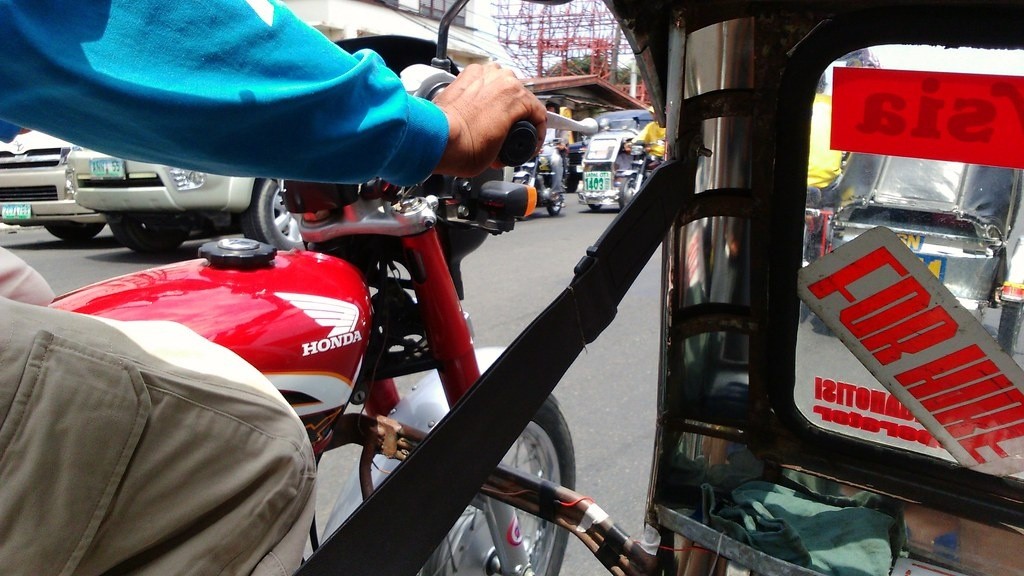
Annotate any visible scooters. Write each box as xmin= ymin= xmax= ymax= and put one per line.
xmin=804 ymin=186 xmax=835 ymax=266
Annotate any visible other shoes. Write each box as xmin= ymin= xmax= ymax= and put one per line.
xmin=551 ymin=192 xmax=559 ymax=201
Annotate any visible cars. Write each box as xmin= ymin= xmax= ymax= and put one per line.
xmin=828 ymin=149 xmax=1017 ymax=332
xmin=1 ymin=129 xmax=108 ymax=244
xmin=63 ymin=135 xmax=312 ymax=255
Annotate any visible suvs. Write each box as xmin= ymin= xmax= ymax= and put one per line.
xmin=564 ymin=109 xmax=656 ymax=194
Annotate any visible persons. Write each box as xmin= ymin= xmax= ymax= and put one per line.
xmin=624 ymin=106 xmax=667 ymax=160
xmin=0 ymin=0 xmax=553 ymax=576
xmin=541 ymin=104 xmax=570 ymax=200
xmin=803 ymin=47 xmax=885 ymax=266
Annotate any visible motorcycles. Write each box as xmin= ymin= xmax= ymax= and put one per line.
xmin=508 ymin=137 xmax=571 ymax=216
xmin=576 ymin=131 xmax=656 ymax=213
xmin=30 ymin=2 xmax=582 ymax=576
